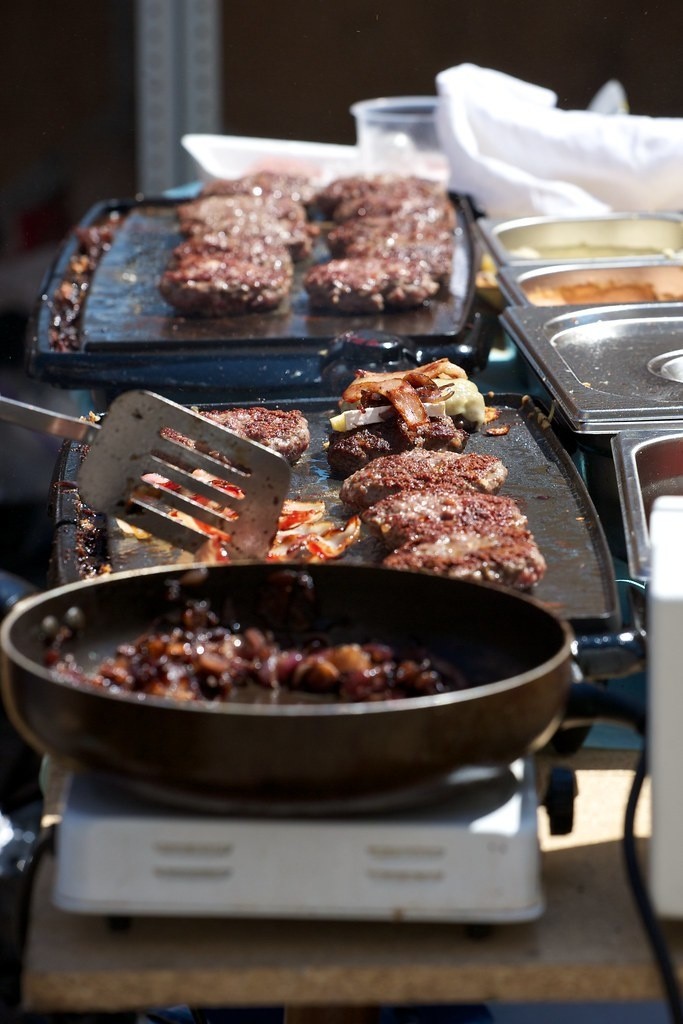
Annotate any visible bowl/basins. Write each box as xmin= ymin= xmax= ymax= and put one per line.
xmin=345 ymin=97 xmax=450 ymax=179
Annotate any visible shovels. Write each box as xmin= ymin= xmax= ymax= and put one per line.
xmin=0 ymin=390 xmax=291 ymax=560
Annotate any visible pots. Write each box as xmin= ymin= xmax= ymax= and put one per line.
xmin=1 ymin=560 xmax=576 ymax=805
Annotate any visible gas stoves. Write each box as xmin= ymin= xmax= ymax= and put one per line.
xmin=23 ymin=757 xmax=582 ymax=932
